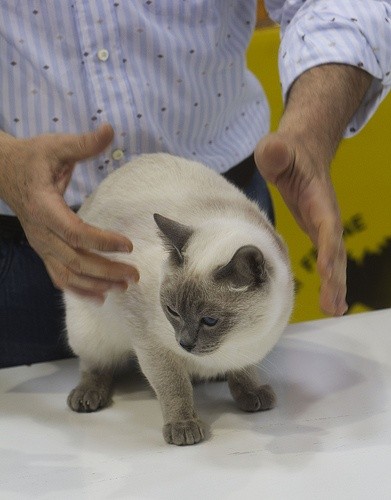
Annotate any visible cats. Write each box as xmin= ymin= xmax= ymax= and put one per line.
xmin=56 ymin=151 xmax=295 ymax=446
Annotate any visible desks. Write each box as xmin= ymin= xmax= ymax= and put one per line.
xmin=0 ymin=305 xmax=391 ymax=500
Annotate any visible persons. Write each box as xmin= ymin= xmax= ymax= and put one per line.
xmin=0 ymin=1 xmax=391 ymax=369
